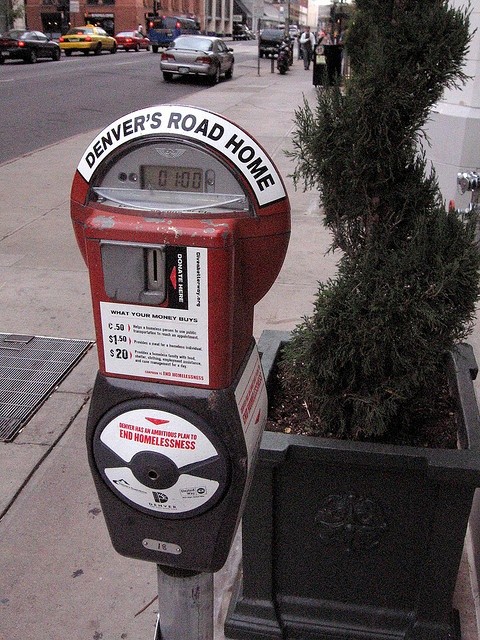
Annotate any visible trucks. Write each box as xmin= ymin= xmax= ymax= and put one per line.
xmin=149 ymin=15 xmax=201 ymax=53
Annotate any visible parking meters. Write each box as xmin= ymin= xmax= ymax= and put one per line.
xmin=71 ymin=105 xmax=290 ymax=640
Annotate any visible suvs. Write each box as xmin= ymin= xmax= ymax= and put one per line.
xmin=258 ymin=29 xmax=295 ymax=58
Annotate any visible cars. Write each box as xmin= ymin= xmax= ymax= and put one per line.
xmin=160 ymin=34 xmax=234 ymax=83
xmin=114 ymin=30 xmax=150 ymax=51
xmin=59 ymin=24 xmax=118 ymax=57
xmin=0 ymin=29 xmax=61 ymax=64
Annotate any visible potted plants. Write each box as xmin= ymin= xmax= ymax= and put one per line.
xmin=222 ymin=0 xmax=478 ymax=640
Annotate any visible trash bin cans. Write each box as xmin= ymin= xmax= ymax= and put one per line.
xmin=312 ymin=44 xmax=342 ymax=87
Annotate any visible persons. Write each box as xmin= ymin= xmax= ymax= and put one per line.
xmin=297 ymin=28 xmax=304 ymax=60
xmin=138 ymin=23 xmax=143 ymax=34
xmin=299 ymin=26 xmax=316 ymax=70
xmin=318 ymin=31 xmax=333 ymax=45
xmin=232 ymin=21 xmax=239 ymax=41
